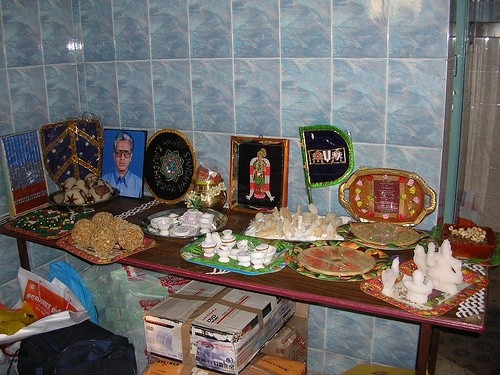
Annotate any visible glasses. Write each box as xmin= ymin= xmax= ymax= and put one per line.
xmin=114 ymin=151 xmax=133 ymax=159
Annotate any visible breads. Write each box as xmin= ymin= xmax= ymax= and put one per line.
xmin=71 ymin=212 xmax=144 ymax=252
xmin=62 ymin=173 xmax=112 ymax=204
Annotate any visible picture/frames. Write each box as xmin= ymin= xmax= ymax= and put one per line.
xmin=230 ymin=135 xmax=290 ymax=215
xmin=101 ymin=129 xmax=147 ymax=200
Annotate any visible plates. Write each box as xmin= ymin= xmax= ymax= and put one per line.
xmin=56 ymin=235 xmax=157 ymax=265
xmin=140 ymin=206 xmax=229 ymax=239
xmin=47 ymin=187 xmax=121 ymax=207
xmin=360 ymin=258 xmax=490 ymax=319
xmin=283 ymin=240 xmax=392 ymax=283
xmin=179 ymin=230 xmax=294 ymax=276
xmin=336 ymin=221 xmax=436 ymax=251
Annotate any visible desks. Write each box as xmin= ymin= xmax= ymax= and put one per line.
xmin=0 ymin=197 xmax=491 ymax=375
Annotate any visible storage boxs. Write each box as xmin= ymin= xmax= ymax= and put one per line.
xmin=140 ymin=280 xmax=310 ymax=375
xmin=340 ymin=363 xmax=415 ymax=375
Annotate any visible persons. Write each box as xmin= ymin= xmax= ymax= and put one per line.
xmin=102 ymin=132 xmax=142 ymax=198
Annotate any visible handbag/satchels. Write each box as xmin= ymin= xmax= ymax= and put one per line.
xmin=7 ymin=319 xmax=138 ymax=375
xmin=0 ymin=261 xmax=99 ymax=345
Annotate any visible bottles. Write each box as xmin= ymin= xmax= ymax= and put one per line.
xmin=192 ymin=181 xmax=227 ymax=210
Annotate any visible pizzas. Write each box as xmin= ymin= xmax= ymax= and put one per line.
xmin=351 ymin=222 xmax=420 ymax=246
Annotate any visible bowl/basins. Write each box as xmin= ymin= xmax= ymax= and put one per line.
xmin=441 ymin=223 xmax=496 ymax=259
